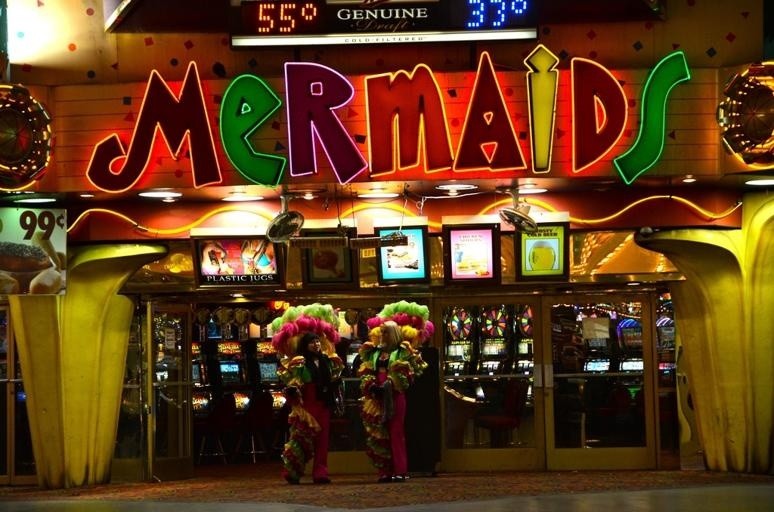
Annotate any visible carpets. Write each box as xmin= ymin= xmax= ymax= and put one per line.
xmin=0 ymin=470 xmax=773 ymax=511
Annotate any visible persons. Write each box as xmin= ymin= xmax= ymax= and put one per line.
xmin=282 ymin=335 xmax=342 ymax=485
xmin=359 ymin=320 xmax=408 ymax=482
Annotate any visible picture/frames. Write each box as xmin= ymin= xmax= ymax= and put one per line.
xmin=441 ymin=222 xmax=503 ymax=286
xmin=373 ymin=225 xmax=432 ymax=286
xmin=298 ymin=227 xmax=362 ymax=290
xmin=514 ymin=221 xmax=570 ymax=286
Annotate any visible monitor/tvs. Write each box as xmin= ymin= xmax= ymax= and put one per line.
xmin=446 ymin=338 xmax=676 ymax=375
xmin=220 ymin=362 xmax=241 ymax=385
xmin=192 ymin=364 xmax=201 ymax=382
xmin=258 ymin=363 xmax=280 ymax=383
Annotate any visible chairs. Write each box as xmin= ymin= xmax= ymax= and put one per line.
xmin=473 ymin=378 xmax=528 ymax=449
xmin=194 ymin=391 xmax=292 ymax=466
xmin=598 ymin=387 xmax=676 ymax=447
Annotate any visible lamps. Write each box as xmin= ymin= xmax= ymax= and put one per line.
xmin=289 ymin=191 xmax=407 ymax=250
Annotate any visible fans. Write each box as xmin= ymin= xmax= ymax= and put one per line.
xmin=267 ymin=195 xmax=304 ymax=243
xmin=494 ymin=188 xmax=538 ymax=236
